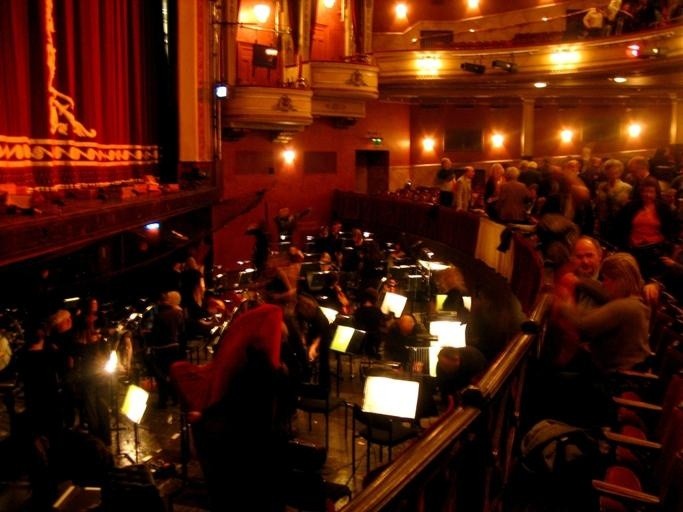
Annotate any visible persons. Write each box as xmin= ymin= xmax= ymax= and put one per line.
xmin=621 ymin=1 xmax=662 ymax=34
xmin=585 ymin=4 xmax=636 ymax=40
xmin=0 ymin=257 xmax=245 ymax=510
xmin=244 ymin=142 xmax=682 ymax=512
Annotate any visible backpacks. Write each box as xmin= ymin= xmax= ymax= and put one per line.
xmin=518 ymin=416 xmax=618 ymax=512
